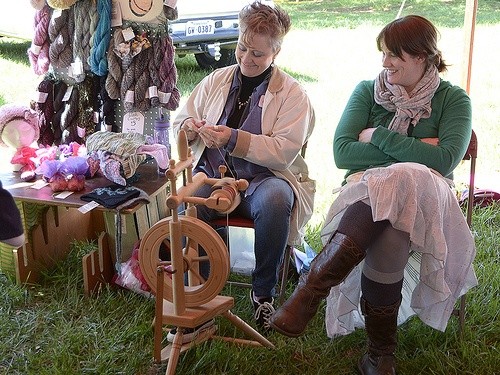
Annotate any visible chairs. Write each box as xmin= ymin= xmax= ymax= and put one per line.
xmin=448 ymin=128 xmax=479 ymax=340
xmin=204 ymin=138 xmax=309 ymax=303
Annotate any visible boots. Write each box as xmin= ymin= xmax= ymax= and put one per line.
xmin=268 ymin=230 xmax=367 ymax=338
xmin=360 ymin=294 xmax=403 ymax=375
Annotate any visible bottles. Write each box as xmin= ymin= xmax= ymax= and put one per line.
xmin=154 ymin=116 xmax=169 ymax=160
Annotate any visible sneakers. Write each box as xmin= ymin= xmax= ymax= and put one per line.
xmin=166 ymin=317 xmax=216 ymax=344
xmin=250 ymin=289 xmax=275 ymax=338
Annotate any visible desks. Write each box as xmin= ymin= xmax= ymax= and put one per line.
xmin=0 ymin=162 xmax=185 ymax=299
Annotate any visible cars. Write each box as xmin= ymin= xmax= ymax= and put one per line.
xmin=0 ymin=0 xmax=274 ymax=77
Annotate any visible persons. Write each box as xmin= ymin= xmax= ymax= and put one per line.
xmin=0 ymin=180 xmax=25 ymax=247
xmin=269 ymin=14 xmax=478 ymax=375
xmin=173 ymin=1 xmax=316 ymax=336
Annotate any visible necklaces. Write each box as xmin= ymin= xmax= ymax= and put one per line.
xmin=238 ymin=93 xmax=251 ymax=110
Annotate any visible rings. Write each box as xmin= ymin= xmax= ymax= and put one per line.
xmin=214 ymin=125 xmax=218 ymax=129
